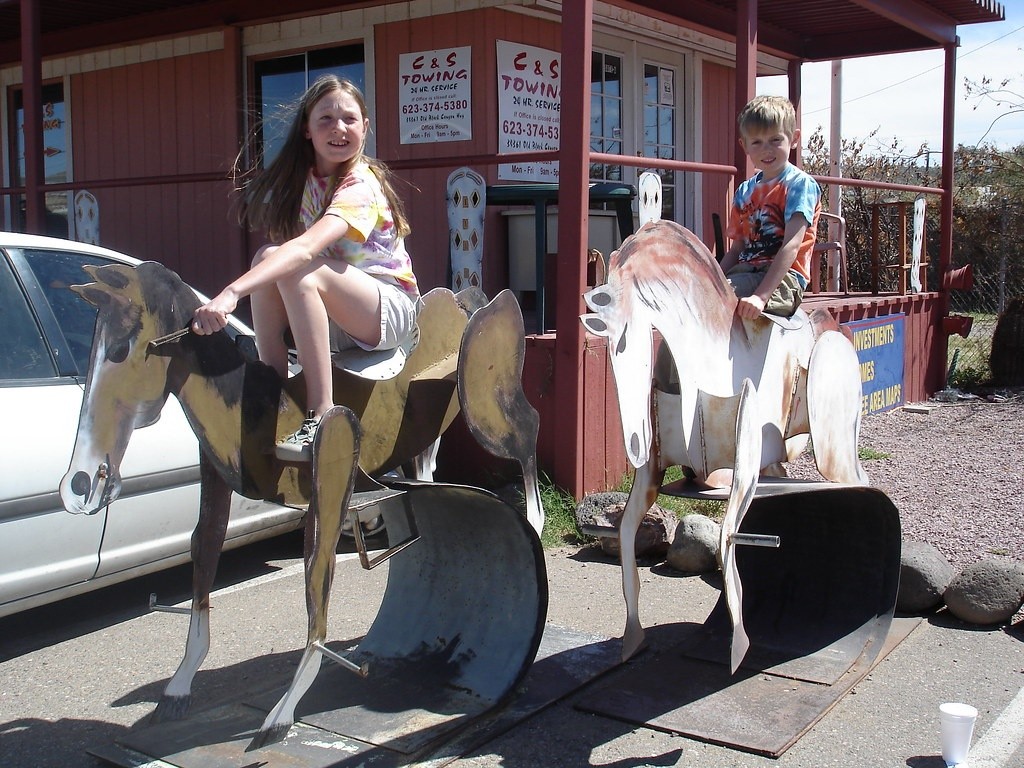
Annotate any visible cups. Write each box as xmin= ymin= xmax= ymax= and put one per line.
xmin=939 ymin=703 xmax=977 ymax=763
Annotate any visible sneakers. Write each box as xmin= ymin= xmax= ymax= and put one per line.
xmin=276 ymin=409 xmax=319 ymax=463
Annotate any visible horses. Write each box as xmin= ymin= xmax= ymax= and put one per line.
xmin=57 ymin=259 xmax=545 ymax=752
xmin=577 ymin=218 xmax=870 ymax=676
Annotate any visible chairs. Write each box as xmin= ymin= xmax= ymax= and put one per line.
xmin=810 ymin=211 xmax=848 ymax=295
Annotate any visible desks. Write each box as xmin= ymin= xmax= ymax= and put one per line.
xmin=485 ymin=181 xmax=634 ymax=320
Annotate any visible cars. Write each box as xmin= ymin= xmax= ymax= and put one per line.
xmin=0 ymin=234 xmax=310 ymax=619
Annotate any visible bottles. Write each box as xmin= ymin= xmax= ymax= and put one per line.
xmin=934 ymin=390 xmax=958 ymax=402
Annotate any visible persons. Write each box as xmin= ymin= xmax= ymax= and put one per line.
xmin=719 ymin=95 xmax=824 ymax=322
xmin=191 ymin=77 xmax=425 ymax=463
